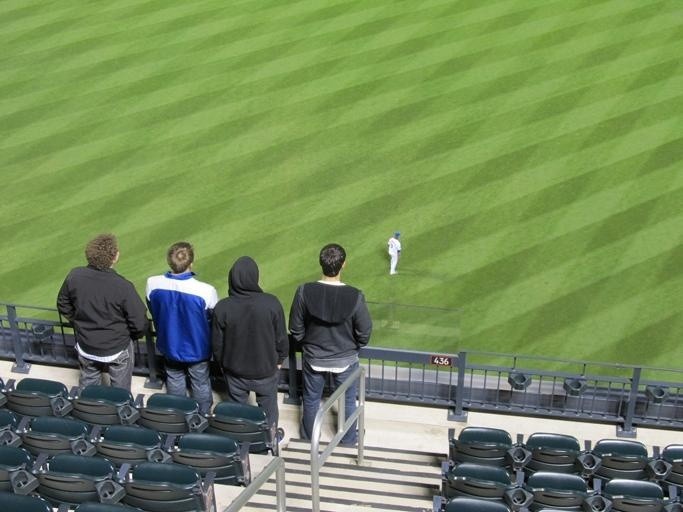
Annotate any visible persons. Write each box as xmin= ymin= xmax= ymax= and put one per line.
xmin=53 ymin=231 xmax=149 ymax=403
xmin=141 ymin=239 xmax=220 ymax=416
xmin=386 ymin=231 xmax=401 ymax=275
xmin=286 ymin=242 xmax=373 ymax=446
xmin=208 ymin=255 xmax=290 ymax=450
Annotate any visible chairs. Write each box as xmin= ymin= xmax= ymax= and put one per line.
xmin=434 ymin=426 xmax=683 ymax=512
xmin=0 ymin=376 xmax=280 ymax=512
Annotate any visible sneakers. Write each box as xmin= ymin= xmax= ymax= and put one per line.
xmin=356 ymin=428 xmax=365 ymax=443
xmin=275 ymin=428 xmax=285 ymax=443
